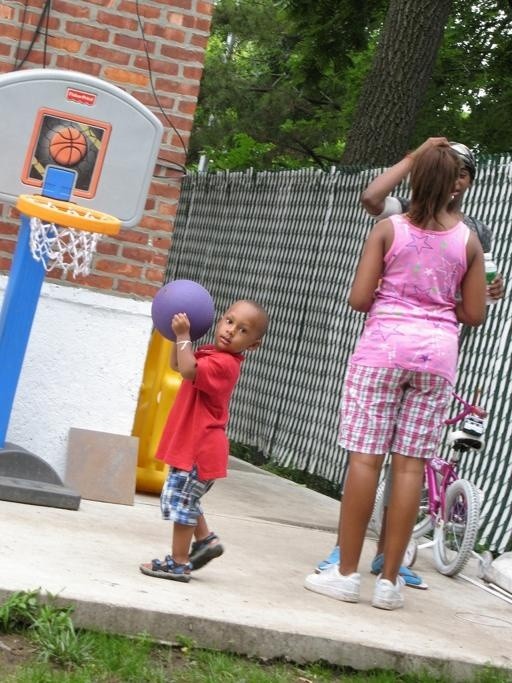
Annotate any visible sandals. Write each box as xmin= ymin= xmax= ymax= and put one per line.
xmin=188 ymin=530 xmax=224 ymax=570
xmin=139 ymin=552 xmax=194 ymax=582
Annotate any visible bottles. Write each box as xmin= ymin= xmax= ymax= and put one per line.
xmin=483 ymin=253 xmax=496 ymax=305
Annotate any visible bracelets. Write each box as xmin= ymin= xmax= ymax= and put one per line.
xmin=176 ymin=341 xmax=192 ymax=351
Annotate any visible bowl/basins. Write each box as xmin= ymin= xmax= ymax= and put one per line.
xmin=446 ymin=141 xmax=475 ymax=168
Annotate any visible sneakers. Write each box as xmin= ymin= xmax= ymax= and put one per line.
xmin=303 ymin=563 xmax=362 ymax=604
xmin=370 ymin=574 xmax=407 ymax=611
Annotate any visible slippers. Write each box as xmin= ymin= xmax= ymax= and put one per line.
xmin=315 ymin=556 xmax=334 ymax=573
xmin=371 ymin=564 xmax=430 ymax=592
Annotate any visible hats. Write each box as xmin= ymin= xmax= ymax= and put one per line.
xmin=448 ymin=141 xmax=480 ymax=172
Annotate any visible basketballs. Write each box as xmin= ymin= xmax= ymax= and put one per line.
xmin=152 ymin=279 xmax=215 ymax=343
xmin=50 ymin=129 xmax=87 ymax=164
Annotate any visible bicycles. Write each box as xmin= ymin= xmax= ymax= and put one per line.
xmin=368 ymin=401 xmax=490 ymax=577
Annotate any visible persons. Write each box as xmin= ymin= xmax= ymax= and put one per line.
xmin=314 ymin=136 xmax=504 ymax=589
xmin=305 ymin=144 xmax=486 ymax=609
xmin=140 ymin=299 xmax=268 ymax=582
